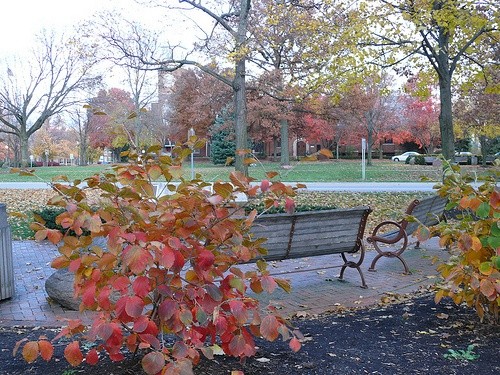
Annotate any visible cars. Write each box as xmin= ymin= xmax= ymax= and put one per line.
xmin=392 ymin=152 xmax=419 ymax=162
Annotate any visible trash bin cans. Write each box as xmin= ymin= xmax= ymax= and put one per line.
xmin=1 ymin=203 xmax=15 ymax=303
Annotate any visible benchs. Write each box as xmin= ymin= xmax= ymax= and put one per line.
xmin=366 ymin=191 xmax=451 ymax=275
xmin=190 ymin=205 xmax=373 ymax=289
